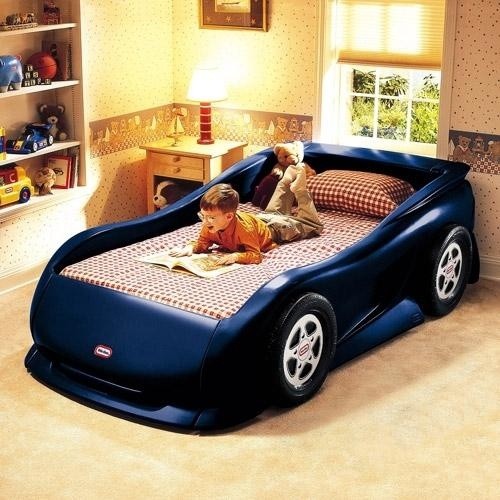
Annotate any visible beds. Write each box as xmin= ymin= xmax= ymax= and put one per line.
xmin=24 ymin=138 xmax=481 ymax=437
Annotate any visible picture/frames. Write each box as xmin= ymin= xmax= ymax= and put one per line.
xmin=40 ymin=154 xmax=72 ymax=189
xmin=196 ymin=0 xmax=269 ymax=33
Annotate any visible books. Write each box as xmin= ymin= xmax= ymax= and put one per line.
xmin=131 ymin=243 xmax=242 ymax=279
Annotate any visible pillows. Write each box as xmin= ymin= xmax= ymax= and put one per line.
xmin=304 ymin=167 xmax=416 ymax=217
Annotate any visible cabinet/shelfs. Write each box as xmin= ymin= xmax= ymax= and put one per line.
xmin=1 ymin=0 xmax=94 ymax=228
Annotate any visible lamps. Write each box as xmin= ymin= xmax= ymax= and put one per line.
xmin=185 ymin=67 xmax=227 ymax=144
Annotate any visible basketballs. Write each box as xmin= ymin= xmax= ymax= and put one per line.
xmin=29 ymin=53 xmax=57 ymax=81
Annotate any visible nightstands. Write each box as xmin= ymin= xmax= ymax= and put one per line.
xmin=139 ymin=135 xmax=247 ymax=216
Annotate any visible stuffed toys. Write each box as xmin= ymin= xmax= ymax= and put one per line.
xmin=251 ymin=171 xmax=282 ymax=211
xmin=270 ymin=139 xmax=317 ymax=180
xmin=152 ymin=179 xmax=195 ymax=211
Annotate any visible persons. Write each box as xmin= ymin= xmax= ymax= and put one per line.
xmin=168 ymin=164 xmax=323 ymax=266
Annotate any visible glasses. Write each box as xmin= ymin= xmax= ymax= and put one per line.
xmin=197 ymin=211 xmax=217 ymax=224
